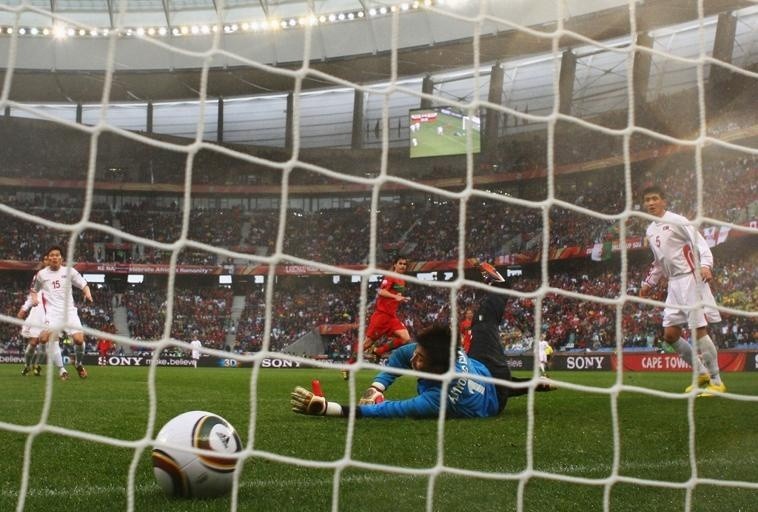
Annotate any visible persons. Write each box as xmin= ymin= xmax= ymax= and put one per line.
xmin=190 ymin=335 xmax=201 ymax=367
xmin=340 ymin=258 xmax=411 ymax=377
xmin=3 ymin=65 xmax=757 ymax=185
xmin=18 ymin=255 xmax=67 ymax=376
xmin=33 ymin=245 xmax=93 ymax=377
xmin=288 ymin=262 xmax=556 ymax=420
xmin=0 ymin=234 xmax=756 ymax=357
xmin=2 ymin=138 xmax=758 ymax=265
xmin=533 ymin=335 xmax=552 ymax=375
xmin=637 ymin=186 xmax=726 ymax=401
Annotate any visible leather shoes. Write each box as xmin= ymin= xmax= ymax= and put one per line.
xmin=357 ymin=382 xmax=386 ymax=405
xmin=480 ymin=265 xmax=505 ymax=283
xmin=290 ymin=379 xmax=342 ymax=416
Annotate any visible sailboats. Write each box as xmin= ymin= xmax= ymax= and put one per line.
xmin=151 ymin=412 xmax=243 ymax=499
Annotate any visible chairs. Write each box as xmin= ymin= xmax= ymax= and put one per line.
xmin=341 ymin=371 xmax=348 ymax=380
xmin=697 ymin=382 xmax=726 ymax=398
xmin=58 ymin=368 xmax=70 ymax=381
xmin=372 ymin=347 xmax=381 ymax=364
xmin=684 ymin=374 xmax=710 ymax=394
xmin=33 ymin=363 xmax=41 ymax=375
xmin=19 ymin=366 xmax=31 ymax=376
xmin=75 ymin=361 xmax=87 ymax=379
